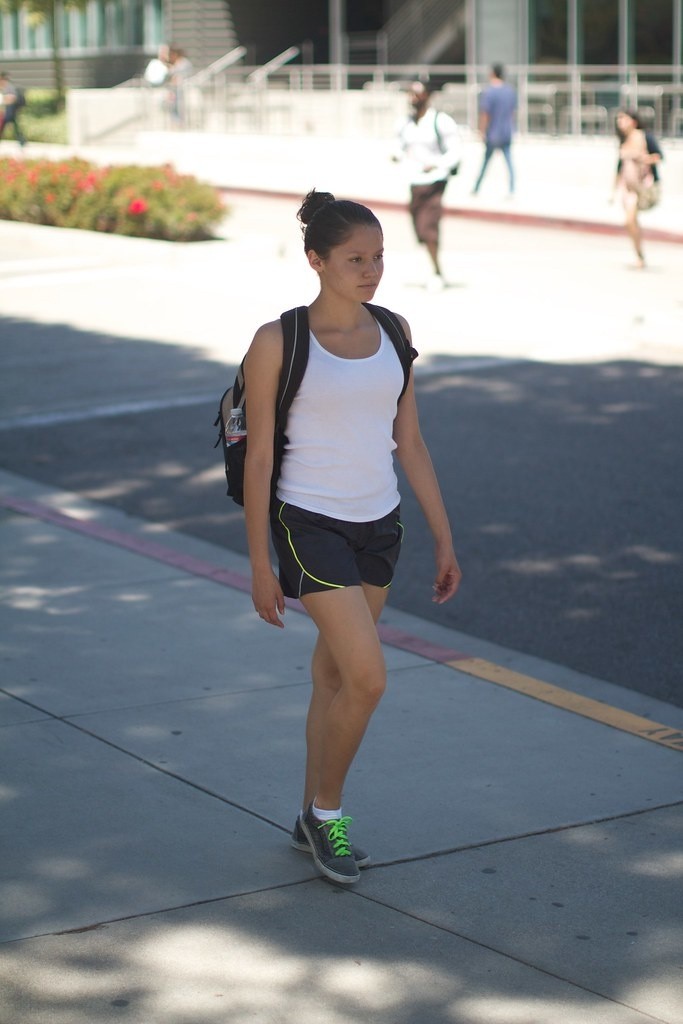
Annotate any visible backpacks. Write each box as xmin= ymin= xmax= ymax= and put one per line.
xmin=213 ymin=300 xmax=420 ymax=508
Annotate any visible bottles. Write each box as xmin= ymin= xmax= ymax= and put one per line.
xmin=225 ymin=408 xmax=247 ymax=447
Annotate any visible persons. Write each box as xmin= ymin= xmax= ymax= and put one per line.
xmin=244 ymin=191 xmax=461 ymax=883
xmin=0 ymin=73 xmax=26 ymax=147
xmin=608 ymin=106 xmax=663 ymax=269
xmin=391 ymin=80 xmax=463 ymax=289
xmin=468 ymin=62 xmax=517 ymax=196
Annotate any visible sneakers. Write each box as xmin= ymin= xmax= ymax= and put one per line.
xmin=290 ymin=814 xmax=370 ymax=868
xmin=300 ymin=796 xmax=361 ymax=883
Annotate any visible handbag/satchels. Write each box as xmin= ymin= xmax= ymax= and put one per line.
xmin=636 ymin=180 xmax=659 ymax=211
